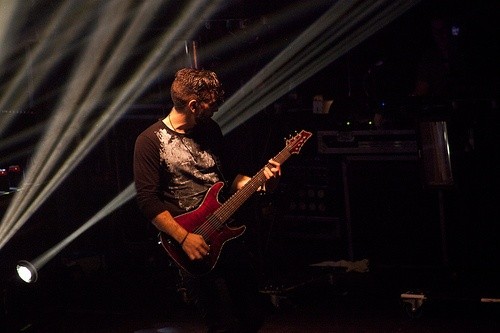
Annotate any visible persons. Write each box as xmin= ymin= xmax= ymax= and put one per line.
xmin=135 ymin=67 xmax=281 ymax=333
xmin=373 ymin=12 xmax=482 ymax=148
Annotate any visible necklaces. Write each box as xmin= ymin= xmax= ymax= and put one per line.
xmin=168 ymin=114 xmax=198 ymax=164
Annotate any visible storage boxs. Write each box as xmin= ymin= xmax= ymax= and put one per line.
xmin=343 ymin=156 xmax=444 ymax=273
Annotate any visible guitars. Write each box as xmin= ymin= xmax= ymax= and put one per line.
xmin=156 ymin=126 xmax=315 ymax=280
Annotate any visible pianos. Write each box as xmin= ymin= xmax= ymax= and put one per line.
xmin=316 ymin=130 xmax=418 ymax=157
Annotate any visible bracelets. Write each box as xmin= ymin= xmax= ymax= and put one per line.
xmin=180 ymin=232 xmax=190 ymax=247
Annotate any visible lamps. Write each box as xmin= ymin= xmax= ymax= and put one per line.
xmin=7 ymin=259 xmax=38 ymax=295
